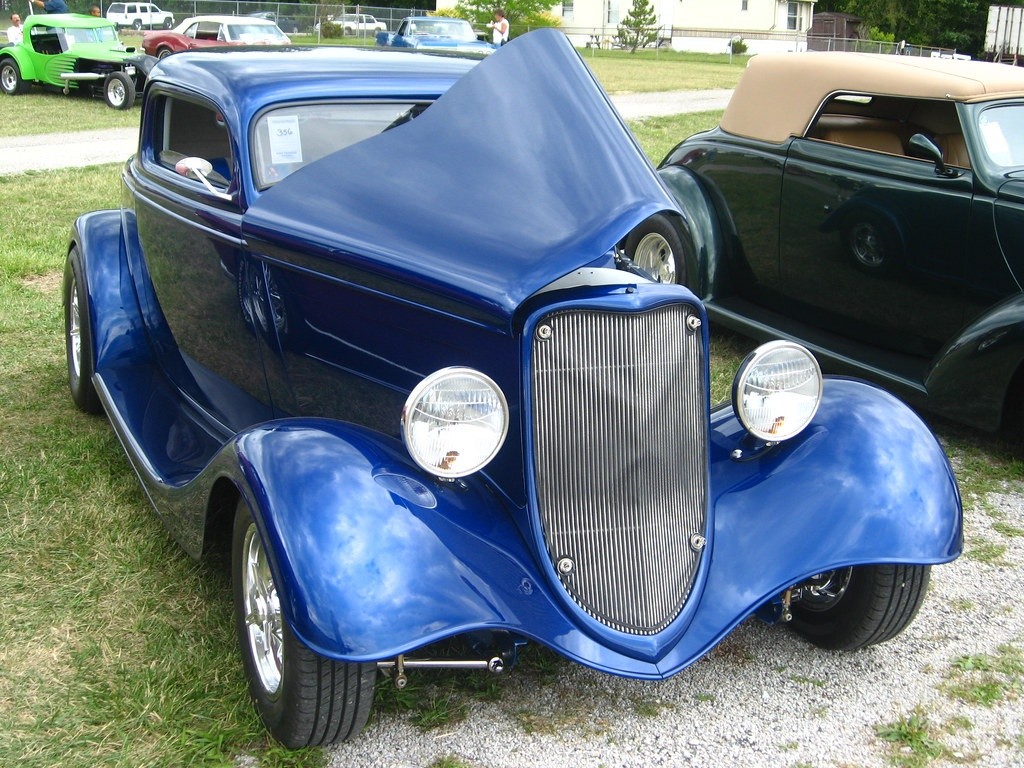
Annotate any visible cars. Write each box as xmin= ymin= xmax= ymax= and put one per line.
xmin=141 ymin=14 xmax=292 ymax=60
xmin=315 ymin=13 xmax=388 ymax=37
xmin=245 ymin=9 xmax=305 ymax=34
xmin=60 ymin=27 xmax=965 ymax=751
xmin=377 ymin=15 xmax=498 ymax=51
xmin=614 ymin=50 xmax=1024 ymax=437
xmin=0 ymin=12 xmax=164 ymax=111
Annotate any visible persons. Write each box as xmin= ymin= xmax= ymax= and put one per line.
xmin=85 ymin=6 xmax=103 ymax=42
xmin=6 ymin=14 xmax=24 ymax=45
xmin=485 ymin=10 xmax=509 ymax=43
xmin=30 ymin=0 xmax=70 ymax=34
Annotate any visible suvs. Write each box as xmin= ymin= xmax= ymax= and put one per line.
xmin=106 ymin=2 xmax=176 ymax=31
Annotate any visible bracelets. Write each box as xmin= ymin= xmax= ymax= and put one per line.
xmin=32 ymin=0 xmax=34 ymax=3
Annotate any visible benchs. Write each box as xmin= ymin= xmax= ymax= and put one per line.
xmin=813 ymin=114 xmax=971 ymax=169
